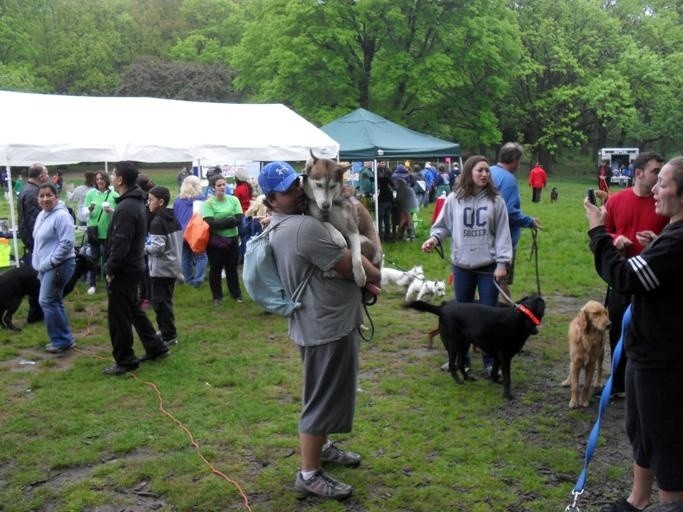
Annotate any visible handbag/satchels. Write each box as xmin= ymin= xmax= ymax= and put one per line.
xmin=183 ymin=213 xmax=210 ymax=253
xmin=87 ymin=226 xmax=98 ymax=243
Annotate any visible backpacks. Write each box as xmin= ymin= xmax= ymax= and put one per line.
xmin=243 ymin=215 xmax=314 ymax=317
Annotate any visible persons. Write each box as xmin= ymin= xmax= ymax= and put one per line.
xmin=339 ymin=143 xmax=547 ymax=379
xmin=583 ymin=152 xmax=682 ymax=511
xmin=257 ymin=158 xmax=382 ymax=499
xmin=0 ymin=143 xmax=258 ymax=375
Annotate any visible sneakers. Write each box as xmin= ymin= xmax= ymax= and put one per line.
xmin=320 ymin=442 xmax=361 ymax=465
xmin=102 ymin=331 xmax=177 ymax=374
xmin=46 ymin=340 xmax=75 ymax=352
xmin=295 ymin=469 xmax=352 ymax=498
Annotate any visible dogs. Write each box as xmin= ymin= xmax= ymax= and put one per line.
xmin=560 ymin=299 xmax=612 ymax=409
xmin=594 ymin=190 xmax=611 ymax=206
xmin=398 ymin=293 xmax=546 ymax=401
xmin=0 ymin=253 xmax=100 ymax=332
xmin=300 ymin=148 xmax=383 ymax=301
xmin=427 ymin=296 xmax=540 ymax=353
xmin=390 ymin=199 xmax=412 ymax=241
xmin=380 ymin=253 xmax=425 ymax=295
xmin=405 ymin=277 xmax=446 ymax=304
xmin=551 ymin=187 xmax=558 ymax=204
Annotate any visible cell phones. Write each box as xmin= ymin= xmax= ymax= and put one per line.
xmin=587 ymin=188 xmax=598 ymax=206
xmin=89 ymin=202 xmax=96 ymax=210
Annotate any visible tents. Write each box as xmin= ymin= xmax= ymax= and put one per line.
xmin=313 ymin=107 xmax=467 ymax=253
xmin=0 ymin=90 xmax=339 ymax=270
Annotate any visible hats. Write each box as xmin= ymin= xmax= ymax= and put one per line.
xmin=258 ymin=161 xmax=307 ymax=193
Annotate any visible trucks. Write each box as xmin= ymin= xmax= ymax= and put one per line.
xmin=596 ymin=147 xmax=641 ymax=186
